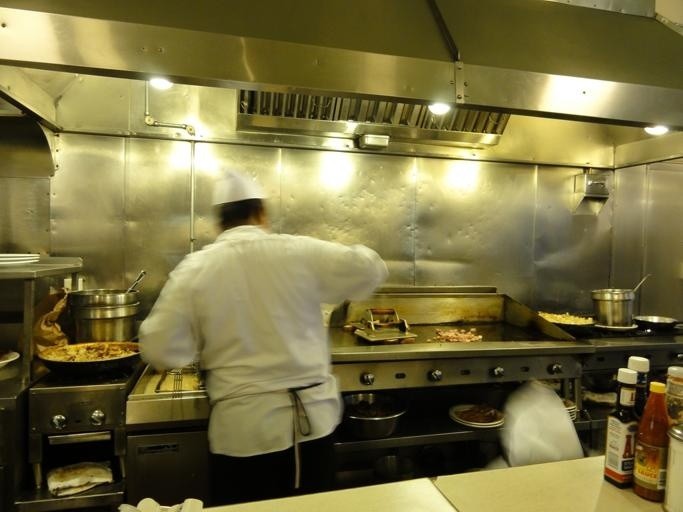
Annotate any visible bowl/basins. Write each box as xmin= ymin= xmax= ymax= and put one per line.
xmin=341 ymin=393 xmax=409 ymax=440
xmin=545 ymin=319 xmax=596 ymax=337
xmin=37 ymin=340 xmax=144 ymax=382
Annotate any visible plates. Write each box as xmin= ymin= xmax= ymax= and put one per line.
xmin=559 ymin=397 xmax=579 ymax=423
xmin=0 ymin=253 xmax=41 ymax=267
xmin=0 ymin=351 xmax=19 ymax=370
xmin=593 ymin=324 xmax=638 ymax=332
xmin=449 ymin=405 xmax=506 ymax=428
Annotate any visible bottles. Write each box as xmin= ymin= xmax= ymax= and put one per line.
xmin=603 ymin=355 xmax=683 ymax=512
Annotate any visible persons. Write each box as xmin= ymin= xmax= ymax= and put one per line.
xmin=137 ymin=175 xmax=391 ymax=505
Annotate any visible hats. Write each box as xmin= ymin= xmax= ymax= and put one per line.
xmin=211 ymin=165 xmax=266 ymax=207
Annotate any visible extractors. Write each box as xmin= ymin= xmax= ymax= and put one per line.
xmin=0 ymin=0 xmax=683 ymax=168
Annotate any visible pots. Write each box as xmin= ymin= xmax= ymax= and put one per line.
xmin=65 ymin=290 xmax=142 ymax=347
xmin=638 ymin=315 xmax=683 ymax=330
